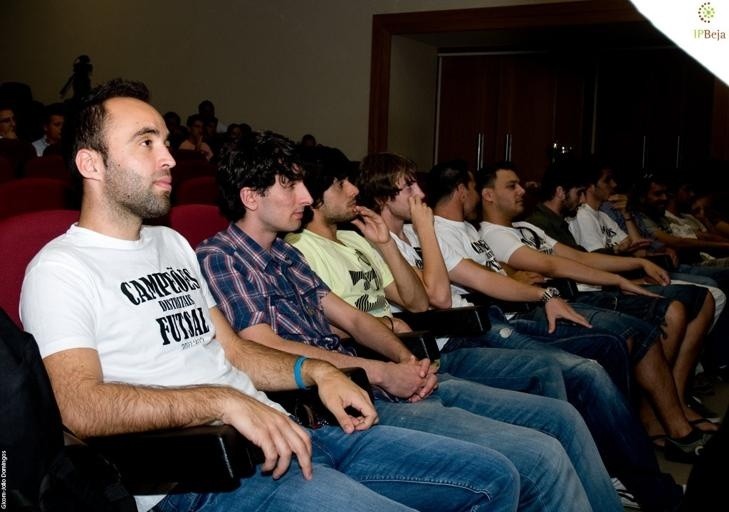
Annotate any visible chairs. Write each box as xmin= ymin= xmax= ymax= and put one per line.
xmin=176 ymin=174 xmax=230 ymax=207
xmin=378 ymin=304 xmax=492 ymax=362
xmin=3 ymin=176 xmax=85 ymax=217
xmin=2 ymin=211 xmax=373 ymax=511
xmin=169 ymin=204 xmax=438 ymax=416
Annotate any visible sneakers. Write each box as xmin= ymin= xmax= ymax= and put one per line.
xmin=608 ymin=478 xmax=686 ymax=511
xmin=662 ymin=434 xmax=706 ymax=465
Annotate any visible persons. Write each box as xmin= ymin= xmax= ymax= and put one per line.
xmin=33 ymin=103 xmax=65 ymax=156
xmin=526 ymin=158 xmax=720 ymax=449
xmin=196 ymin=133 xmax=623 ymax=512
xmin=637 ymin=168 xmax=729 ymax=289
xmin=199 ymin=100 xmax=215 ymax=119
xmin=478 ymin=164 xmax=709 ymax=461
xmin=429 ymin=160 xmax=690 ymax=511
xmin=605 ymin=162 xmax=725 ymax=343
xmin=18 ymin=78 xmax=521 ymax=512
xmin=177 ymin=114 xmax=211 ymax=164
xmin=358 ymin=152 xmax=643 ymax=512
xmin=205 ymin=116 xmax=218 ymax=153
xmin=283 ymin=145 xmax=639 ymax=507
xmin=301 ymin=134 xmax=315 ymax=148
xmin=226 ymin=124 xmax=240 ymax=152
xmin=566 ymin=158 xmax=721 ymax=426
xmin=0 ymin=107 xmax=17 ymax=140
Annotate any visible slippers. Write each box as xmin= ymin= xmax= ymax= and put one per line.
xmin=687 ymin=418 xmax=722 ymax=433
xmin=646 ymin=434 xmax=670 ymax=451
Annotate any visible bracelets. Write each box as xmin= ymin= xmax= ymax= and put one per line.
xmin=293 ymin=354 xmax=310 ymax=391
xmin=540 ymin=286 xmax=561 ymax=303
